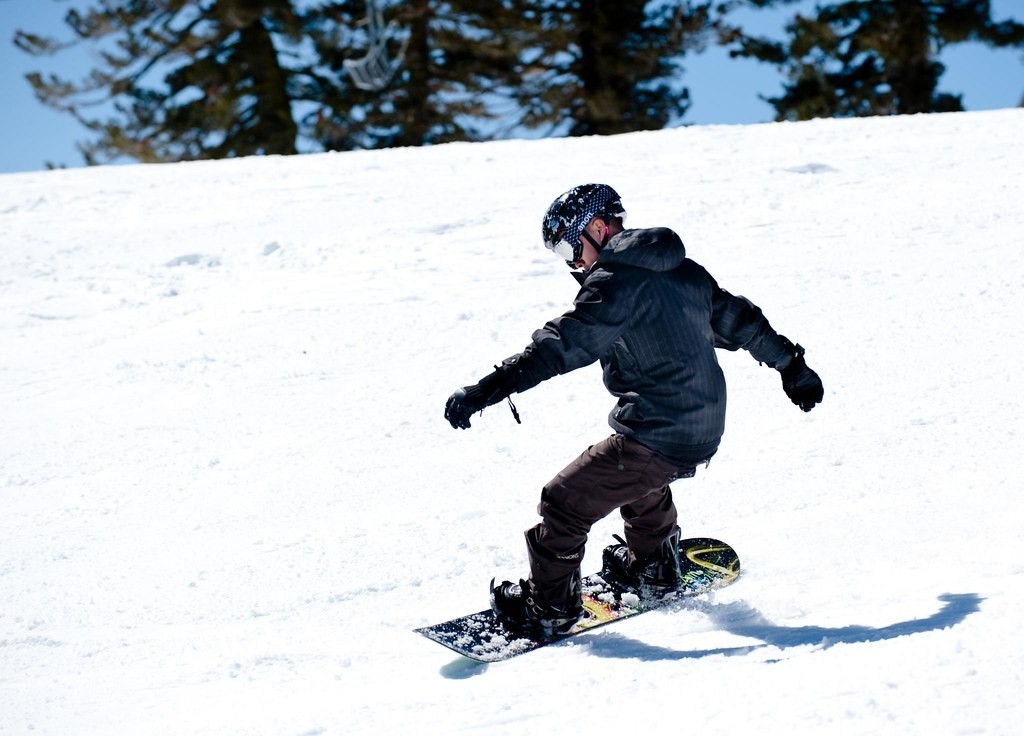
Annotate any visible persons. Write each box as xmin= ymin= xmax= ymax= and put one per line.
xmin=442 ymin=183 xmax=824 ymax=631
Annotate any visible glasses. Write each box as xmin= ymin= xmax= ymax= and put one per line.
xmin=552 ymin=185 xmax=620 ymax=261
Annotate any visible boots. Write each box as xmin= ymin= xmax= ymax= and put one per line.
xmin=489 ymin=530 xmax=586 ymax=626
xmin=603 ymin=526 xmax=683 ymax=594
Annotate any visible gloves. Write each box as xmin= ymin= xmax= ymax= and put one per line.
xmin=446 ymin=392 xmax=480 ymax=428
xmin=779 ymin=356 xmax=824 ymax=411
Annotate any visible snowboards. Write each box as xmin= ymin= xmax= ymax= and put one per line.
xmin=412 ymin=537 xmax=741 ymax=664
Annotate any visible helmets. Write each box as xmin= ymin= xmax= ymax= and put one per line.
xmin=544 ymin=182 xmax=626 ymax=248
